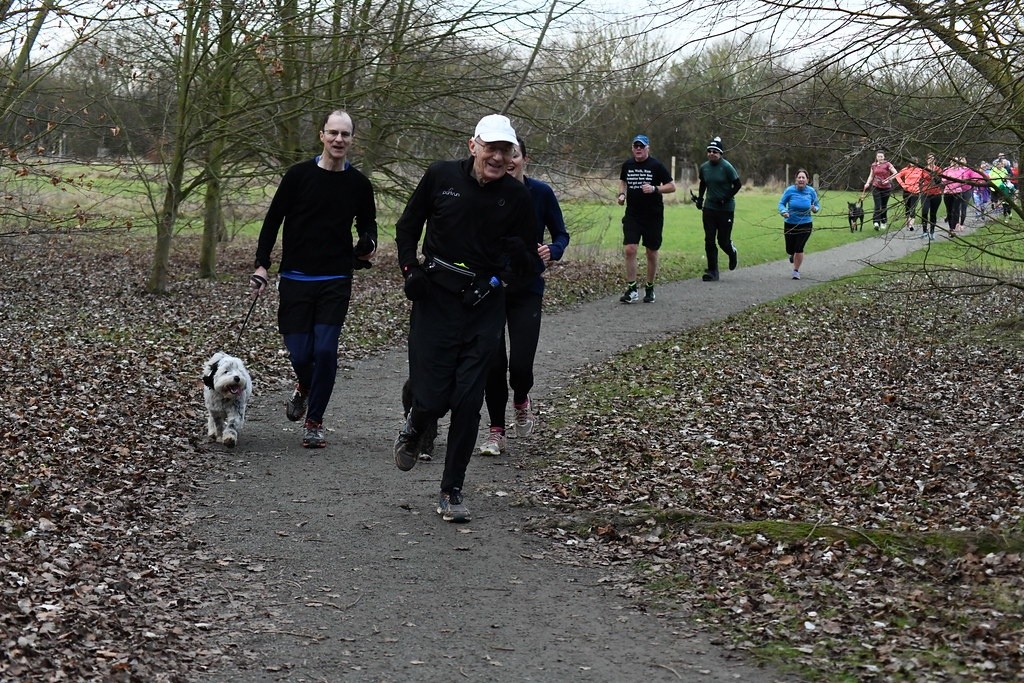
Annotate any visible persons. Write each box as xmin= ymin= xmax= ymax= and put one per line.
xmin=252 ymin=110 xmax=377 ymax=448
xmin=618 ymin=135 xmax=676 ymax=304
xmin=392 ymin=112 xmax=539 ymax=522
xmin=478 ymin=138 xmax=570 ymax=456
xmin=919 ymin=152 xmax=942 ymax=239
xmin=942 ymin=153 xmax=1019 ymax=237
xmin=778 ymin=170 xmax=819 ymax=279
xmin=864 ymin=151 xmax=898 ymax=231
xmin=896 ymin=156 xmax=930 ymax=230
xmin=696 ymin=136 xmax=741 ymax=281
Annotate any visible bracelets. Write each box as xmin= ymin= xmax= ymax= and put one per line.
xmin=619 ymin=193 xmax=625 ymax=196
xmin=654 ymin=186 xmax=658 ymax=192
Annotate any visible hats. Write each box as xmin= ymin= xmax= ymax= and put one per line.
xmin=632 ymin=135 xmax=649 ymax=146
xmin=707 ymin=136 xmax=724 ymax=155
xmin=473 ymin=114 xmax=520 ymax=146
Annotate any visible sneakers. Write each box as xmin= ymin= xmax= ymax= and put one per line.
xmin=286 ymin=383 xmax=309 ymax=422
xmin=513 ymin=394 xmax=534 ymax=439
xmin=702 ymin=271 xmax=719 ymax=281
xmin=480 ymin=427 xmax=507 ymax=455
xmin=619 ymin=289 xmax=639 ymax=303
xmin=437 ymin=487 xmax=472 ymax=522
xmin=643 ymin=289 xmax=656 ymax=303
xmin=728 ymin=249 xmax=738 ymax=270
xmin=792 ymin=271 xmax=800 ymax=280
xmin=303 ymin=416 xmax=326 ymax=448
xmin=789 ymin=255 xmax=793 ymax=263
xmin=392 ymin=407 xmax=419 ymax=471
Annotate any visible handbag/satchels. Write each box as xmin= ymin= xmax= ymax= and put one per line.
xmin=418 ymin=257 xmax=506 ymax=311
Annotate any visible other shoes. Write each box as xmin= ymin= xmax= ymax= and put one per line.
xmin=881 ymin=223 xmax=887 ymax=229
xmin=873 ymin=222 xmax=879 ymax=230
xmin=908 ymin=204 xmax=1013 ymax=238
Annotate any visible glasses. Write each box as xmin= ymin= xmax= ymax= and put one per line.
xmin=633 ymin=144 xmax=645 ymax=149
xmin=475 ymin=139 xmax=516 ymax=157
xmin=707 ymin=149 xmax=717 ymax=154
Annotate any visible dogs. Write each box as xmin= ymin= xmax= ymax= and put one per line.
xmin=202 ymin=350 xmax=252 ymax=448
xmin=847 ymin=200 xmax=865 ymax=233
